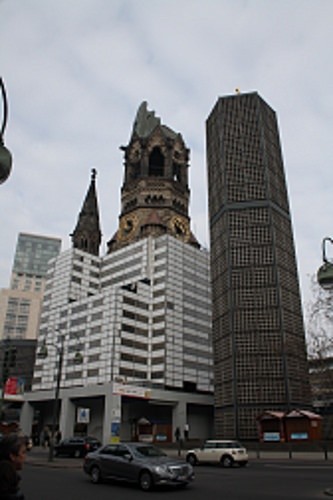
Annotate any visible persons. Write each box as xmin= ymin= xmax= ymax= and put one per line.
xmin=0 ymin=433 xmax=29 ymax=500
xmin=173 ymin=425 xmax=181 ymax=443
xmin=182 ymin=422 xmax=191 ymax=441
xmin=2 ymin=409 xmax=66 ymax=461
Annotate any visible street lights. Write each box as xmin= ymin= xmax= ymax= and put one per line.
xmin=37 ymin=327 xmax=83 ymax=462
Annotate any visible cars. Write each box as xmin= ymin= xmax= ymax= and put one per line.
xmin=84 ymin=441 xmax=196 ymax=490
xmin=54 ymin=437 xmax=100 ymax=459
xmin=187 ymin=440 xmax=249 ymax=468
